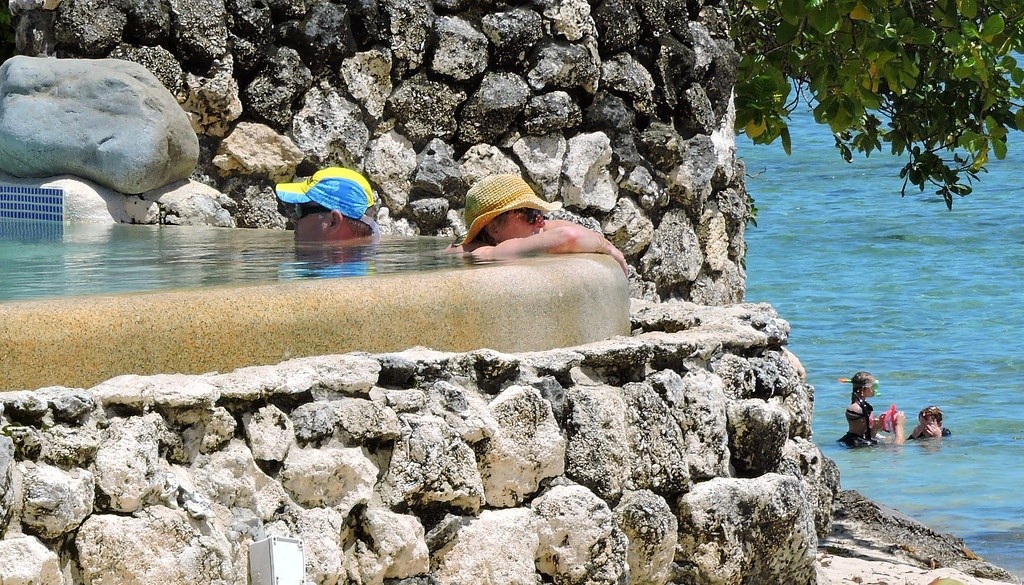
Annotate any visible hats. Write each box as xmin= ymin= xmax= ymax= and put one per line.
xmin=451 ymin=174 xmax=562 ymax=246
xmin=275 ymin=167 xmax=375 ymax=219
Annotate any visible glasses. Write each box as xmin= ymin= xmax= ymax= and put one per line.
xmin=507 ymin=209 xmax=543 ymax=225
xmin=853 ymin=380 xmax=879 ymax=392
xmin=294 ymin=203 xmax=331 ymax=220
xmin=881 ymin=404 xmax=897 ymax=433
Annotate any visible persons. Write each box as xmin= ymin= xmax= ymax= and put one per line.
xmin=906 ymin=405 xmax=951 ymax=441
xmin=838 ymin=373 xmax=905 ymax=448
xmin=450 ymin=171 xmax=630 ymax=277
xmin=269 ymin=169 xmax=376 ymax=252
